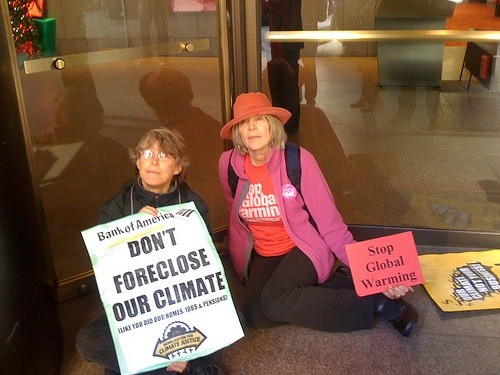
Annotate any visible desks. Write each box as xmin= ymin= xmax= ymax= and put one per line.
xmin=459 ymin=28 xmax=500 ymax=95
xmin=374 ymin=0 xmax=456 ymax=89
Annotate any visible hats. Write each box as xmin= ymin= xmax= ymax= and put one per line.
xmin=139 ymin=68 xmax=193 ymax=108
xmin=219 ymin=91 xmax=292 ymax=140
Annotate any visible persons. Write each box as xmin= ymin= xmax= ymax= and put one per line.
xmin=267 ymin=0 xmax=304 ymax=132
xmin=74 ymin=128 xmax=224 ymax=375
xmin=128 ymin=68 xmax=232 ymax=236
xmin=347 ymin=67 xmax=380 ymax=114
xmin=373 ymin=0 xmax=456 ymax=122
xmin=137 ymin=0 xmax=173 ymax=72
xmin=218 ymin=92 xmax=420 ymax=338
xmin=30 ymin=0 xmax=104 ymax=146
xmin=298 ymin=0 xmax=328 ymax=105
xmin=26 ymin=87 xmax=140 ymax=275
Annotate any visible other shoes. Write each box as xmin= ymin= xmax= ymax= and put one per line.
xmin=191 ymin=356 xmax=223 ymax=375
xmin=382 ymin=293 xmax=418 ymax=338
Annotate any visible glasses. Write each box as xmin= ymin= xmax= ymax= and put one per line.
xmin=138 ymin=149 xmax=176 ymax=161
